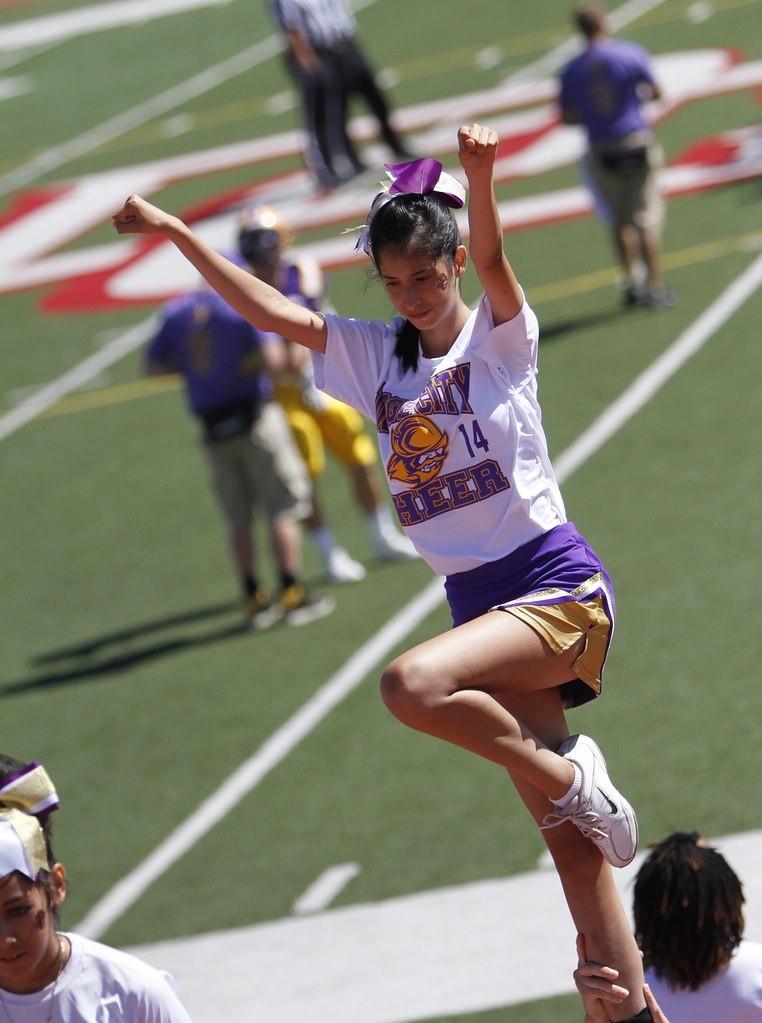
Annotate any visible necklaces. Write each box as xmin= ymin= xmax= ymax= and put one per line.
xmin=0 ymin=935 xmax=64 ymax=1023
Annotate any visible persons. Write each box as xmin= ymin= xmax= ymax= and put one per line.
xmin=558 ymin=5 xmax=670 ymax=312
xmin=573 ymin=932 xmax=670 ymax=1023
xmin=113 ymin=124 xmax=654 ymax=1023
xmin=624 ymin=829 xmax=762 ymax=1023
xmin=275 ymin=0 xmax=420 ymax=192
xmin=144 ymin=204 xmax=422 ymax=629
xmin=0 ymin=753 xmax=192 ymax=1023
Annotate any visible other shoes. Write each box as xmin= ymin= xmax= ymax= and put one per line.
xmin=373 ymin=529 xmax=424 ymax=559
xmin=623 ymin=286 xmax=642 ymax=309
xmin=246 ymin=583 xmax=288 ymax=628
xmin=322 ymin=550 xmax=368 ymax=585
xmin=643 ymin=286 xmax=674 ymax=306
xmin=286 ymin=590 xmax=336 ymax=626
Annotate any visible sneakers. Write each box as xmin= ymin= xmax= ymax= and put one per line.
xmin=541 ymin=731 xmax=638 ymax=867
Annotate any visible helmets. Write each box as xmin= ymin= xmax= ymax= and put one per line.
xmin=235 ymin=205 xmax=294 ymax=261
